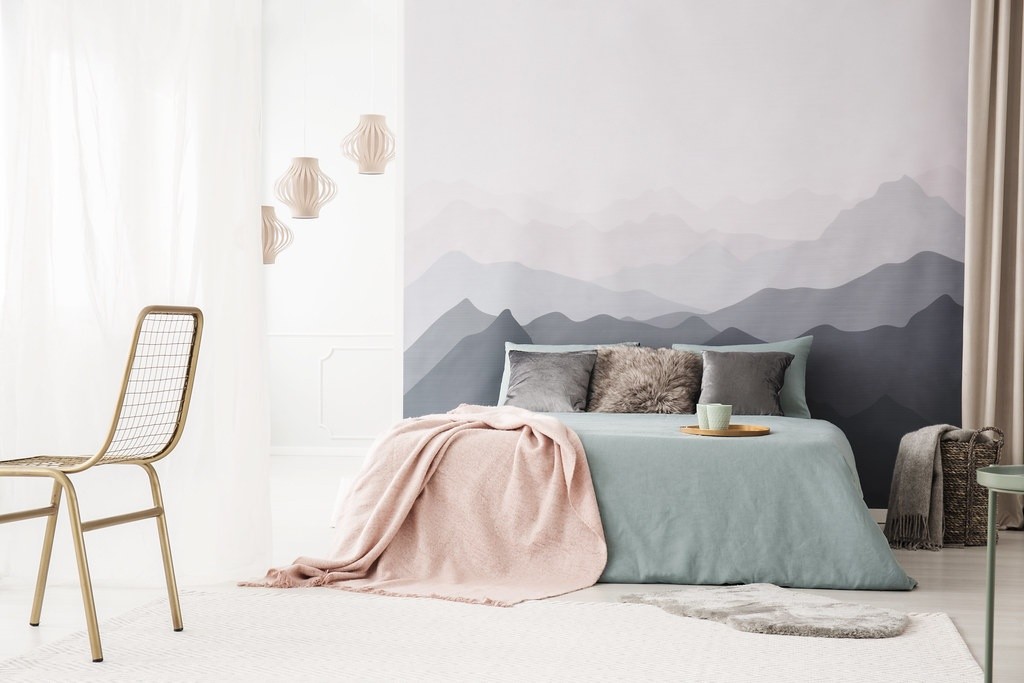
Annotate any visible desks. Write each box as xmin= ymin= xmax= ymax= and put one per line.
xmin=977 ymin=464 xmax=1024 ymax=683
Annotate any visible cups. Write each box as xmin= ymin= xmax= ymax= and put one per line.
xmin=707 ymin=405 xmax=732 ymax=430
xmin=696 ymin=404 xmax=722 ymax=429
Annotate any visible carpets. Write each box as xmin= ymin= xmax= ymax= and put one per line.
xmin=620 ymin=583 xmax=908 ymax=639
xmin=1 ymin=601 xmax=984 ymax=681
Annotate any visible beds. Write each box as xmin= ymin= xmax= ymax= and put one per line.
xmin=329 ymin=335 xmax=918 ymax=591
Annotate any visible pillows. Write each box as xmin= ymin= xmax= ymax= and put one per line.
xmin=672 ymin=335 xmax=814 ymax=419
xmin=586 ymin=346 xmax=703 ymax=414
xmin=497 ymin=341 xmax=640 ymax=408
xmin=504 ymin=349 xmax=598 ymax=412
xmin=698 ymin=351 xmax=795 ymax=416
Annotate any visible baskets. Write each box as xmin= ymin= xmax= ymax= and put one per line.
xmin=939 ymin=425 xmax=1004 ymax=547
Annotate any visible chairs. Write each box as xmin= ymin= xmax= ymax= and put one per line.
xmin=0 ymin=306 xmax=203 ymax=664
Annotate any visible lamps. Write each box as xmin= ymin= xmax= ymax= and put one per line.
xmin=261 ymin=113 xmax=396 ymax=265
